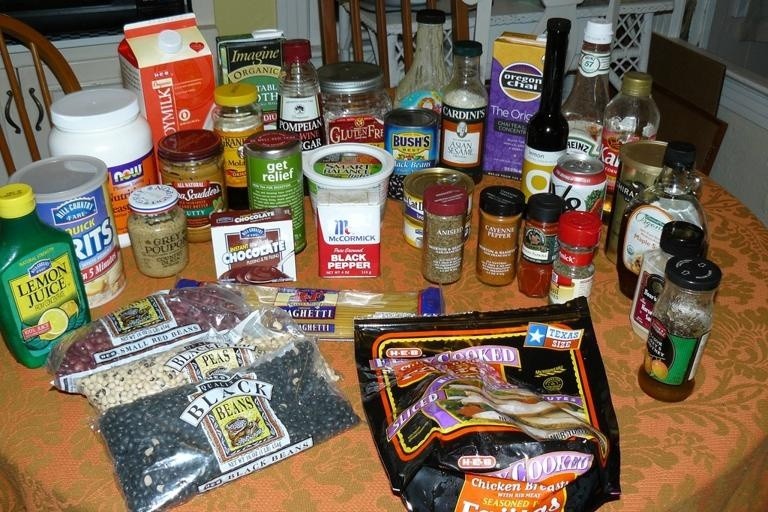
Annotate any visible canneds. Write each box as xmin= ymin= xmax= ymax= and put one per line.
xmin=638 ymin=255 xmax=722 ymax=403
xmin=318 ymin=61 xmax=393 ymax=148
xmin=550 ymin=151 xmax=608 ymax=223
xmin=127 ymin=185 xmax=190 ymax=278
xmin=243 ymin=130 xmax=307 ymax=255
xmin=212 ymin=82 xmax=264 ymax=210
xmin=157 ymin=129 xmax=228 ymax=243
xmin=403 ymin=168 xmax=474 ymax=250
xmin=384 ymin=106 xmax=441 ymax=201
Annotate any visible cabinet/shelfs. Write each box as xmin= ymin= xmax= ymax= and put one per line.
xmin=0 ymin=41 xmax=123 ymax=180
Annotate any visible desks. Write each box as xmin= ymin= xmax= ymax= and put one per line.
xmin=338 ymin=2 xmax=674 ymax=106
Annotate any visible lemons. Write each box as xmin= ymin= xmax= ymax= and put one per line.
xmin=37 ymin=307 xmax=69 ymax=340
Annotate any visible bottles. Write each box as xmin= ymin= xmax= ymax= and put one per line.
xmin=0 ymin=178 xmax=88 ymax=368
xmin=616 ymin=138 xmax=706 ymax=305
xmin=121 ymin=37 xmax=319 ymax=279
xmin=624 ymin=219 xmax=724 ymax=402
xmin=560 ymin=14 xmax=620 ymax=158
xmin=422 ymin=175 xmax=516 ymax=289
xmin=597 ymin=70 xmax=661 ymax=225
xmin=519 ymin=188 xmax=595 ymax=309
xmin=389 ymin=8 xmax=488 ymax=186
xmin=519 ymin=16 xmax=569 ymax=200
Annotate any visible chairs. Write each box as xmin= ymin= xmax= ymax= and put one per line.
xmin=317 ymin=0 xmax=470 ymax=88
xmin=1 ymin=13 xmax=83 ymax=175
